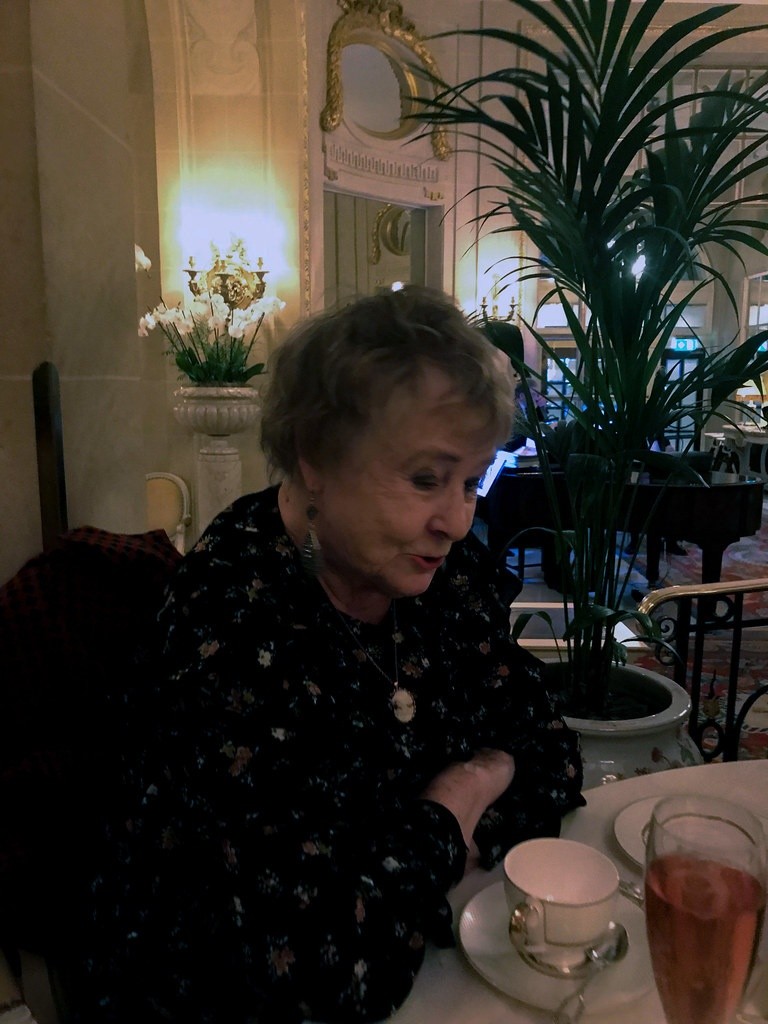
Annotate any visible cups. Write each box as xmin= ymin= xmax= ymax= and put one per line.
xmin=644 ymin=794 xmax=768 ymax=1023
xmin=502 ymin=838 xmax=619 ymax=977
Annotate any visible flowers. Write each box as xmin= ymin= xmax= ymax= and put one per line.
xmin=133 ymin=240 xmax=284 ymax=386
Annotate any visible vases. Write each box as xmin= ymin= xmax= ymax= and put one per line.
xmin=171 ymin=386 xmax=262 ymax=437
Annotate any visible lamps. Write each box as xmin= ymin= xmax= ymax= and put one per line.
xmin=481 ymin=276 xmax=518 ymax=325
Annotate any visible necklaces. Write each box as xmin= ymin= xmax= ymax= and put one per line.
xmin=331 ymin=596 xmax=416 ymax=722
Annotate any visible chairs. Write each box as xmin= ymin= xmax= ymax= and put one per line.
xmin=147 ymin=472 xmax=192 ymax=555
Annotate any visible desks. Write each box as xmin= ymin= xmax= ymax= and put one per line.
xmin=722 ymin=424 xmax=768 ymax=487
xmin=378 ymin=760 xmax=768 ymax=1024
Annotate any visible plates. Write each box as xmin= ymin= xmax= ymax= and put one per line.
xmin=612 ymin=795 xmax=665 ymax=867
xmin=458 ymin=882 xmax=656 ymax=1015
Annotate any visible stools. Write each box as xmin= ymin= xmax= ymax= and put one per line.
xmin=509 ymin=600 xmax=653 ymax=667
xmin=705 ymin=432 xmax=739 ymax=475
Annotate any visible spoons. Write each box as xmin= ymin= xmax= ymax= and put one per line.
xmin=553 ymin=922 xmax=630 ymax=1024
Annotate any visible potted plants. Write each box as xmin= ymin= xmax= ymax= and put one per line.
xmin=392 ymin=1 xmax=768 ymax=785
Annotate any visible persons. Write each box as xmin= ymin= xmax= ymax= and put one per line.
xmin=48 ymin=284 xmax=586 ymax=1024
